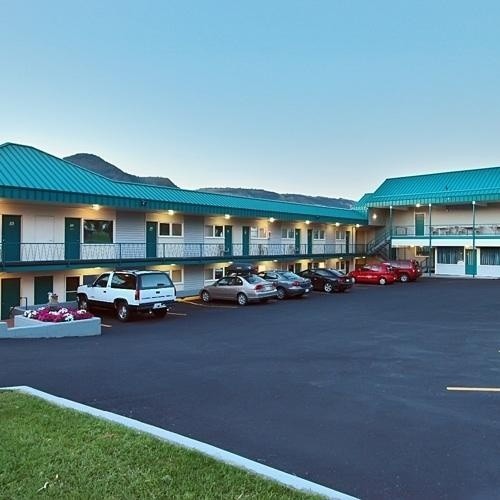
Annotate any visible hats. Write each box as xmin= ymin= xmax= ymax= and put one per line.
xmin=198 ymin=258 xmax=421 ymax=306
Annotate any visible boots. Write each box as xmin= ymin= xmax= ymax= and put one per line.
xmin=75 ymin=269 xmax=178 ymax=322
